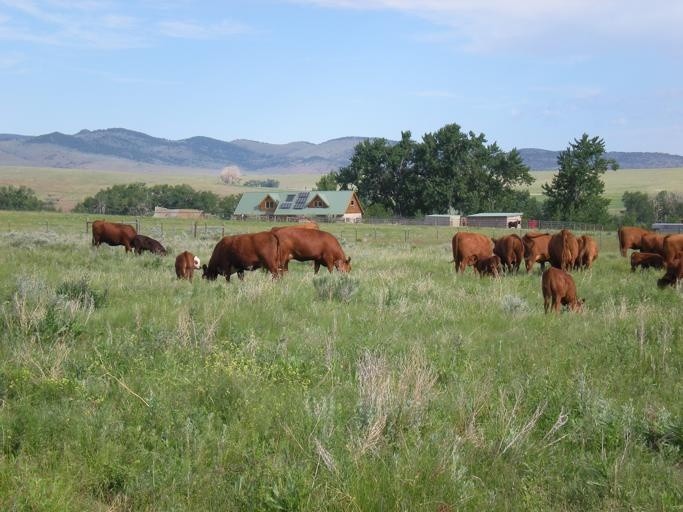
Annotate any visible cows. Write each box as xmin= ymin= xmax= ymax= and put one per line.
xmin=448 ymin=231 xmax=598 ymax=278
xmin=175 ymin=251 xmax=202 ymax=284
xmin=130 ymin=235 xmax=169 ymax=257
xmin=92 ymin=220 xmax=138 ymax=254
xmin=201 ymin=222 xmax=352 ymax=284
xmin=542 ymin=267 xmax=585 ymax=316
xmin=617 ymin=225 xmax=683 ymax=288
